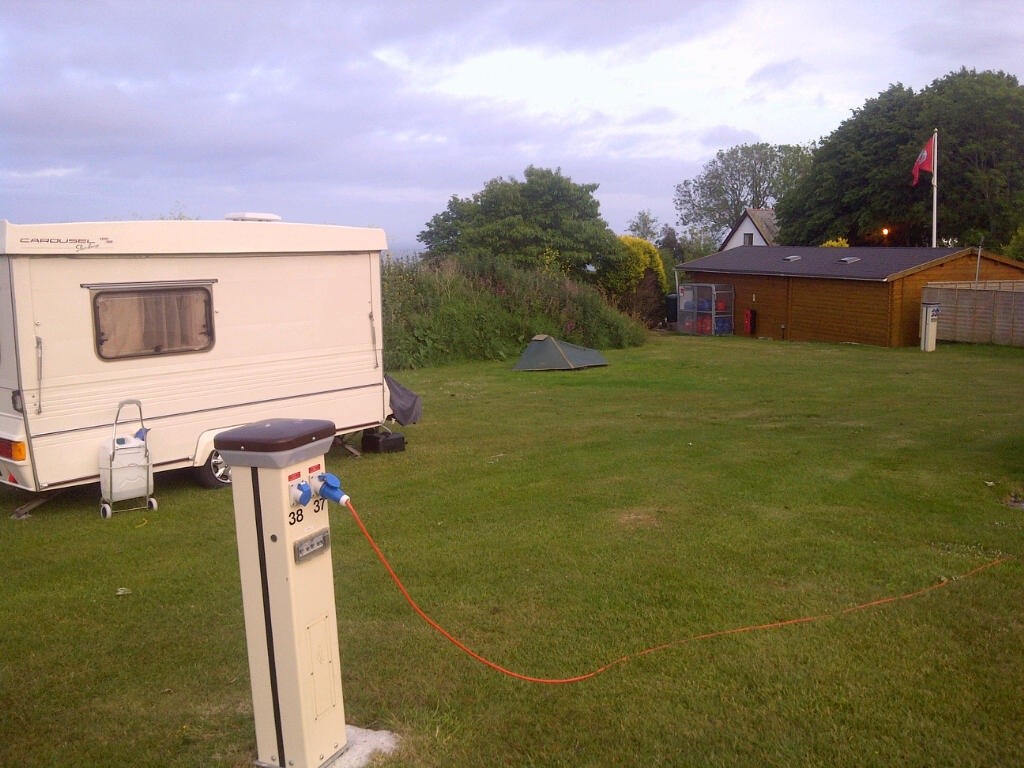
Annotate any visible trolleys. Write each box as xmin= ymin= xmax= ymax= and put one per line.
xmin=100 ymin=399 xmax=159 ymax=520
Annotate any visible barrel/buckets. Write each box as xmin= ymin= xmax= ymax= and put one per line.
xmin=682 ymin=298 xmax=731 ymax=334
xmin=99 ymin=436 xmax=154 ymax=504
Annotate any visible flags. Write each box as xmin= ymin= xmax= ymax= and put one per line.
xmin=912 ymin=135 xmax=933 ymax=185
